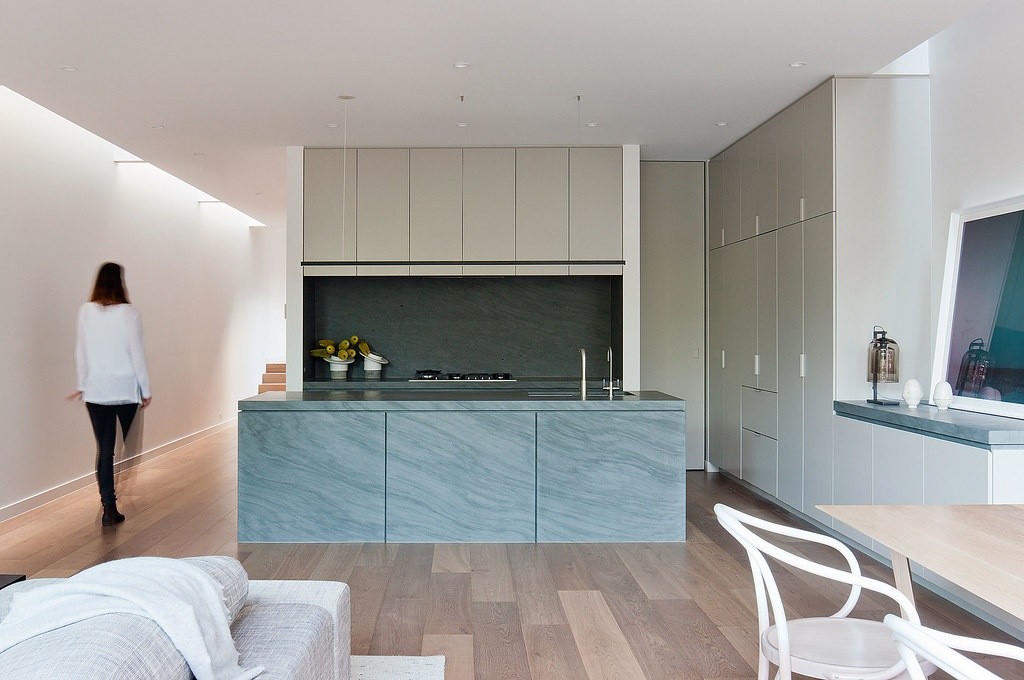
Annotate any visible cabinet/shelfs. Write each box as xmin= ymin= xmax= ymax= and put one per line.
xmin=703 ymin=71 xmax=933 ymax=534
xmin=836 ymin=398 xmax=1024 ymax=621
xmin=304 ymin=145 xmax=625 ymax=275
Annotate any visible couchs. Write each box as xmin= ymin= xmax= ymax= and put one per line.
xmin=0 ymin=555 xmax=352 ymax=680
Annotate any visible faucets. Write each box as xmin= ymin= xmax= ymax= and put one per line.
xmin=578 ymin=348 xmax=588 ymax=398
xmin=606 ymin=347 xmax=614 ymax=399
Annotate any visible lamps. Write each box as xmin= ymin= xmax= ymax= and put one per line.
xmin=868 ymin=325 xmax=900 ymax=406
xmin=956 ymin=337 xmax=997 ymax=396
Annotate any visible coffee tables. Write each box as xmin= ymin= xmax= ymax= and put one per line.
xmin=0 ymin=574 xmax=26 ymax=590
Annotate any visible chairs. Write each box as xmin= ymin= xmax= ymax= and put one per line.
xmin=714 ymin=502 xmax=1024 ymax=680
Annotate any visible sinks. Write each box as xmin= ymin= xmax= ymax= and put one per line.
xmin=526 ymin=390 xmax=636 ymax=399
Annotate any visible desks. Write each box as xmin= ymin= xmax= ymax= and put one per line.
xmin=817 ymin=504 xmax=1024 ymax=643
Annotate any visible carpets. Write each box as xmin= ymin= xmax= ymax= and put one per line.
xmin=351 ymin=655 xmax=446 ymax=680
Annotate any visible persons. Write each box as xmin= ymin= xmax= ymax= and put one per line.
xmin=66 ymin=263 xmax=152 ymax=527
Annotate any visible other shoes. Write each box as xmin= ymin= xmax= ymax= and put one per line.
xmin=101 ymin=499 xmax=124 ymax=526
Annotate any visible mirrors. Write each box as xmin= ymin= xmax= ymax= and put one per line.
xmin=927 ymin=194 xmax=1024 ymax=420
